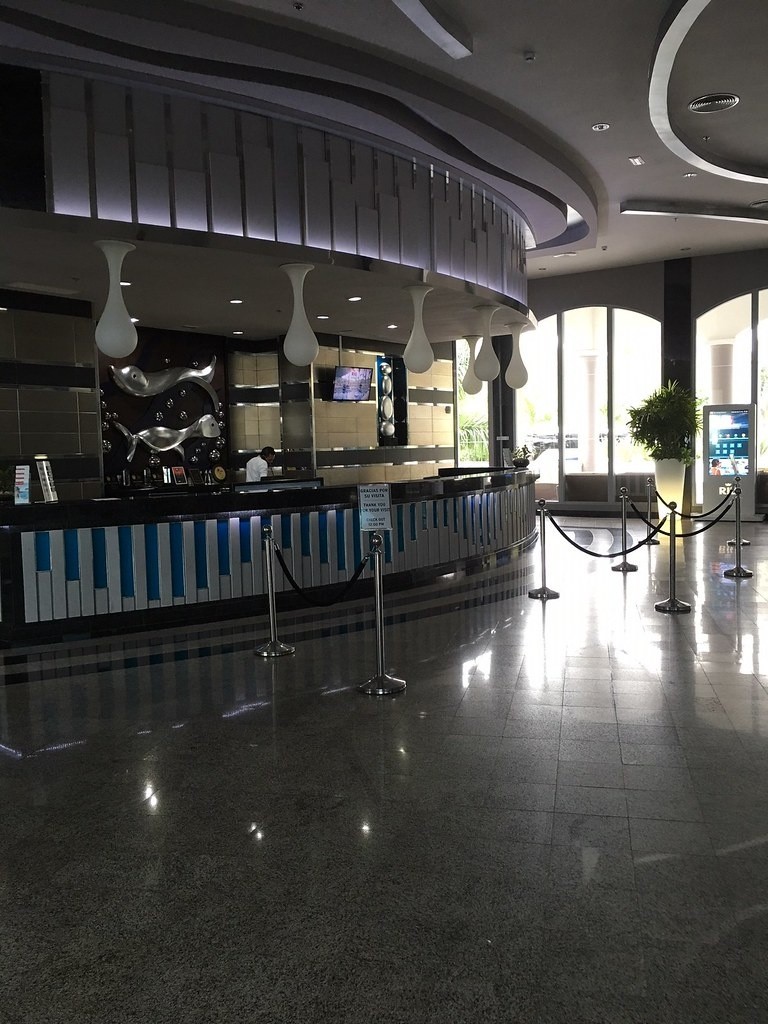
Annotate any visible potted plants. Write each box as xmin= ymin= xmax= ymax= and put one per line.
xmin=626 ymin=380 xmax=707 ymax=521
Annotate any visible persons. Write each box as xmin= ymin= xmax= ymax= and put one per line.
xmin=246 ymin=447 xmax=275 ymax=482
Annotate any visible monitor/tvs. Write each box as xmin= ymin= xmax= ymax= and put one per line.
xmin=708 ymin=410 xmax=749 ymax=476
xmin=331 ymin=366 xmax=373 ymax=402
